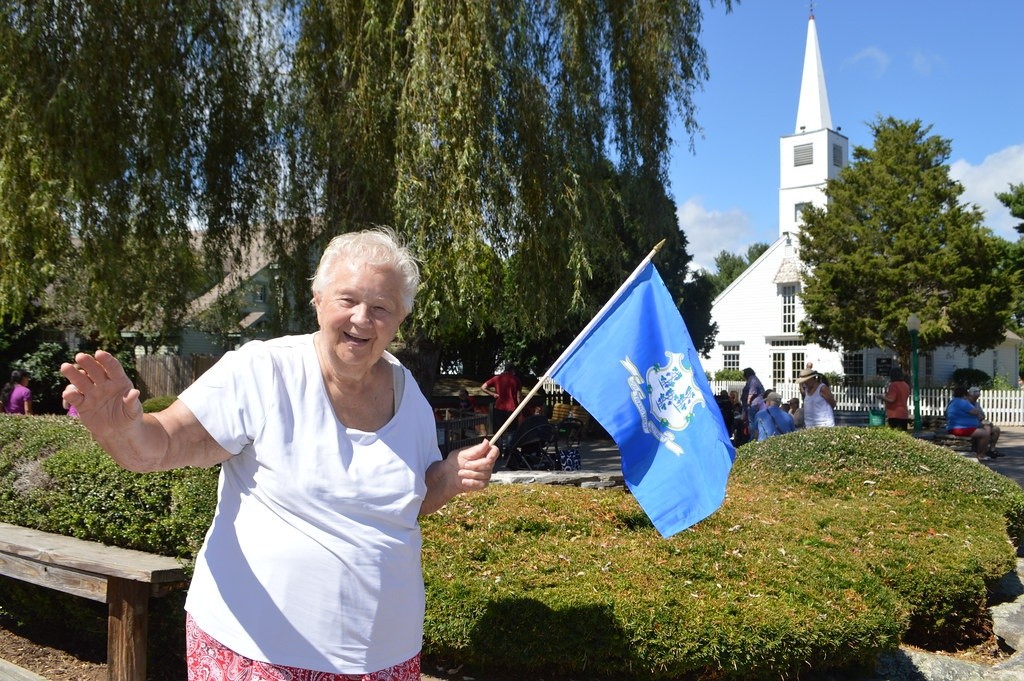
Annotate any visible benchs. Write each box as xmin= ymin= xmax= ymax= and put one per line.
xmin=833 ymin=410 xmax=870 ymax=428
xmin=934 ymin=430 xmax=973 ymax=441
xmin=0 ymin=521 xmax=185 ymax=681
xmin=547 ymin=403 xmax=591 ymax=432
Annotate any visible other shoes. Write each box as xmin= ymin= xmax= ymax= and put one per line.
xmin=978 ymin=456 xmax=991 ymax=461
xmin=992 ymin=450 xmax=1006 ymax=456
xmin=986 ymin=452 xmax=996 ymax=458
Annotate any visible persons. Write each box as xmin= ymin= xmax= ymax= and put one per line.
xmin=1 ymin=370 xmax=34 ymax=415
xmin=63 ymin=368 xmax=88 ymax=418
xmin=945 ymin=386 xmax=1005 ymax=461
xmin=721 ymin=361 xmax=838 ymax=443
xmin=876 ymin=366 xmax=911 ymax=432
xmin=62 ymin=221 xmax=503 ymax=680
xmin=480 ymin=360 xmax=523 ymax=460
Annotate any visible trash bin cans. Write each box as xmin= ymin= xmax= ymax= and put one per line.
xmin=870 ymin=410 xmax=886 ymax=427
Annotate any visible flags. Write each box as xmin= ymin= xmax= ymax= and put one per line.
xmin=552 ymin=260 xmax=735 ymax=542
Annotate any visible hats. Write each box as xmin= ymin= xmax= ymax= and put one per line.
xmin=969 ymin=386 xmax=980 ymax=396
xmin=760 ymin=392 xmax=781 ymax=402
xmin=795 ymin=369 xmax=818 ymax=383
xmin=788 ymin=398 xmax=799 ymax=403
